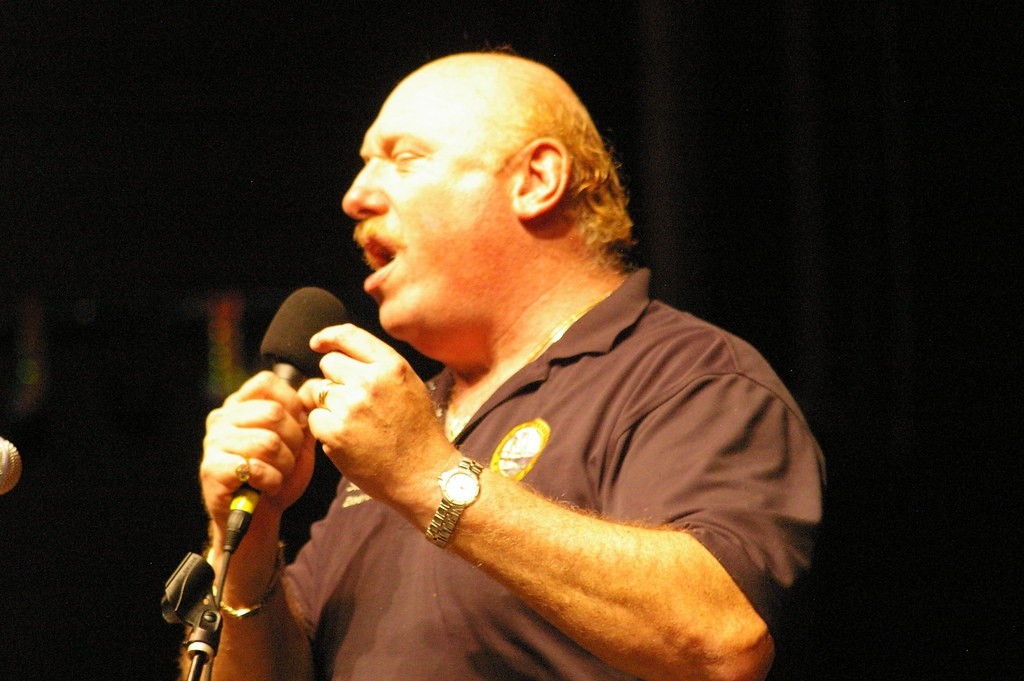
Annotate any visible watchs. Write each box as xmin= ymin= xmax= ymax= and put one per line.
xmin=424 ymin=455 xmax=486 ymax=549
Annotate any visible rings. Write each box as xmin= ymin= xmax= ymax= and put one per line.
xmin=312 ymin=377 xmax=333 ymax=409
xmin=235 ymin=457 xmax=252 ymax=483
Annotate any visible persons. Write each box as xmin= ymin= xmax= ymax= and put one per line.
xmin=175 ymin=49 xmax=836 ymax=681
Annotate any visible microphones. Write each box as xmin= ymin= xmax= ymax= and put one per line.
xmin=222 ymin=285 xmax=346 ymax=552
